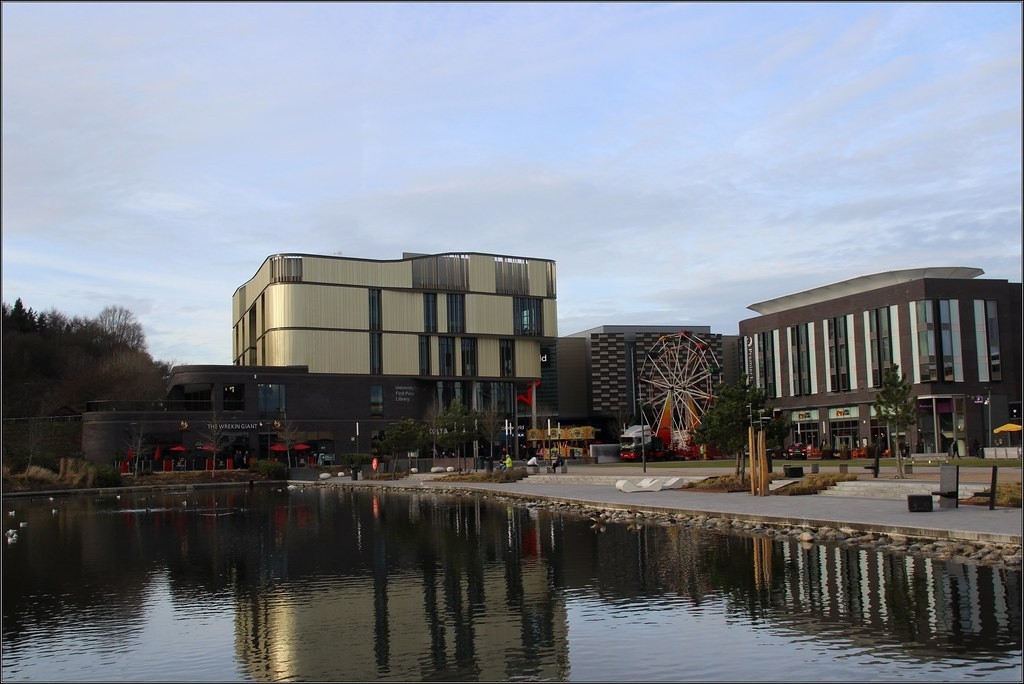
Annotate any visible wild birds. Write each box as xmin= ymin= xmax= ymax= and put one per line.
xmin=49 ymin=497 xmax=54 ymax=500
xmin=20 ymin=521 xmax=28 ymax=525
xmin=5 ymin=529 xmax=19 ymax=545
xmin=52 ymin=509 xmax=58 ymax=513
xmin=182 ymin=500 xmax=186 ymax=503
xmin=8 ymin=510 xmax=15 ymax=514
xmin=117 ymin=495 xmax=120 ymax=498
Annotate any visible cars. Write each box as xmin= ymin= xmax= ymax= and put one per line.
xmin=784 ymin=442 xmax=807 ymax=459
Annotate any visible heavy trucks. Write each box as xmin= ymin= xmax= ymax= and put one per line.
xmin=619 ymin=425 xmax=729 ymax=462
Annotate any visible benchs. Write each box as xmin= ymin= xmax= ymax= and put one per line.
xmin=903 ymin=462 xmax=940 ymax=473
xmin=839 ymin=463 xmax=874 ymax=474
xmin=526 ymin=464 xmax=541 ymax=474
xmin=782 ymin=462 xmax=819 ymax=474
xmin=546 ymin=465 xmax=567 ymax=474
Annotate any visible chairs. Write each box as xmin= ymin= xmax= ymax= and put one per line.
xmin=808 ymin=446 xmax=891 ymax=459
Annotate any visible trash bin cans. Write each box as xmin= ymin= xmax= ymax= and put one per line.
xmin=351 ymin=467 xmax=357 ymax=480
xmin=477 ymin=456 xmax=484 ymax=469
xmin=485 ymin=456 xmax=493 ymax=473
xmin=765 ymin=449 xmax=773 ymax=473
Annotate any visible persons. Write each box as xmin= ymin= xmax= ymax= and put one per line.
xmin=526 ymin=454 xmax=540 ymax=466
xmin=233 ymin=448 xmax=278 ymax=476
xmin=551 ymin=453 xmax=564 ymax=472
xmin=900 ymin=440 xmax=910 ymax=458
xmin=972 ymin=438 xmax=982 ymax=459
xmin=952 ymin=440 xmax=961 ymax=459
xmin=431 ymin=444 xmax=513 ymax=472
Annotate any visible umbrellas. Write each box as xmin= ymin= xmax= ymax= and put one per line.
xmin=169 ymin=444 xmax=187 ymax=470
xmin=198 ymin=444 xmax=221 ymax=471
xmin=993 ymin=423 xmax=1022 ymax=446
xmin=292 ymin=443 xmax=310 ymax=466
xmin=268 ymin=444 xmax=288 ymax=462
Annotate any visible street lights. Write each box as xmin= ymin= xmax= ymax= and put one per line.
xmin=984 ymin=386 xmax=992 ymax=449
